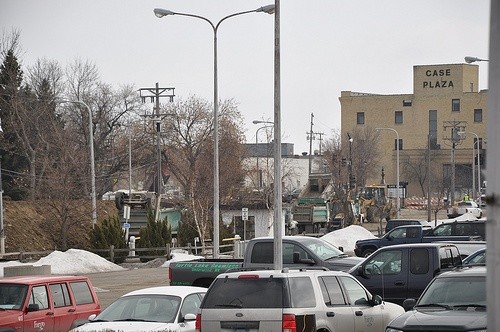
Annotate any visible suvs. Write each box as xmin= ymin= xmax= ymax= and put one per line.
xmin=195 ymin=265 xmax=405 ymax=332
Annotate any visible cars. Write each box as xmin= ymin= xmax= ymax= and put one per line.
xmin=384 ymin=263 xmax=488 ymax=332
xmin=344 ymin=200 xmax=487 ymax=267
xmin=0 ymin=275 xmax=101 ymax=332
xmin=68 ymin=285 xmax=209 ymax=332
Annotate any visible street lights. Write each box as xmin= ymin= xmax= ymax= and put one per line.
xmin=456 ymin=131 xmax=481 ymax=209
xmin=55 ymin=99 xmax=97 ymax=230
xmin=374 ymin=127 xmax=400 ymax=220
xmin=153 ymin=3 xmax=275 ymax=259
xmin=346 ymin=132 xmax=353 ymax=192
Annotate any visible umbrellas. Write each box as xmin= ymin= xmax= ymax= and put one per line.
xmin=290 ymin=221 xmax=299 ymax=226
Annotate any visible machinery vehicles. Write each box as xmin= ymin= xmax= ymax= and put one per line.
xmin=114 ymin=190 xmax=184 ymax=262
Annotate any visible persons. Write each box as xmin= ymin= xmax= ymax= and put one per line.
xmin=287 ymin=220 xmax=300 ymax=236
xmin=462 ymin=192 xmax=472 ymax=204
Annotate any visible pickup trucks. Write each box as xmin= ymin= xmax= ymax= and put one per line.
xmin=167 ymin=234 xmax=387 ymax=288
xmin=354 ymin=224 xmax=483 ymax=259
xmin=345 ymin=242 xmax=487 ymax=312
